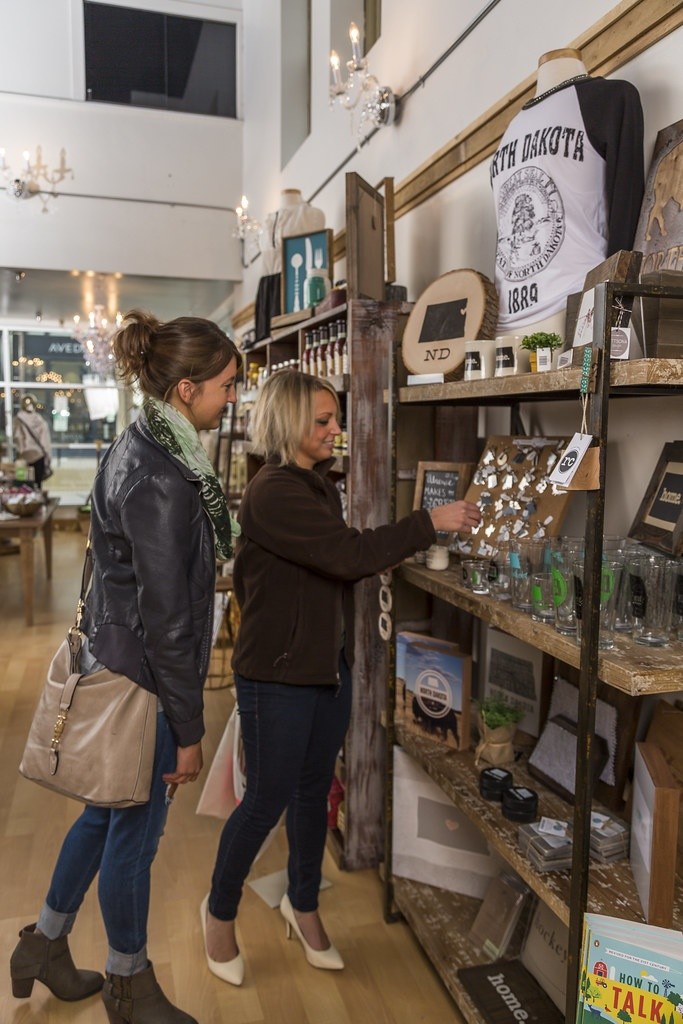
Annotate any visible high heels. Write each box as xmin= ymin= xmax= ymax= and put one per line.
xmin=199 ymin=894 xmax=245 ymax=985
xmin=279 ymin=893 xmax=342 ymax=970
xmin=10 ymin=919 xmax=109 ymax=1001
xmin=101 ymin=963 xmax=200 ymax=1024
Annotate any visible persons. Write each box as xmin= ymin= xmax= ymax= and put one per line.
xmin=14 ymin=397 xmax=50 ymax=490
xmin=198 ymin=370 xmax=481 ymax=985
xmin=255 ymin=188 xmax=324 ymax=342
xmin=493 ymin=48 xmax=646 ymax=377
xmin=11 ymin=315 xmax=243 ymax=1024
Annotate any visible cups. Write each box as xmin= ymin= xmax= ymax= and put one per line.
xmin=462 ymin=341 xmax=497 ymax=381
xmin=417 ymin=502 xmax=683 ymax=674
xmin=492 ymin=334 xmax=533 ymax=376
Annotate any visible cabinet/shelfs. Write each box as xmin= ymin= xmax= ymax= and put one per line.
xmin=379 ymin=357 xmax=683 ymax=1024
xmin=241 ymin=304 xmax=379 ymax=869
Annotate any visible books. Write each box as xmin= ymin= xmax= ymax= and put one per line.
xmin=394 ymin=631 xmax=471 ymax=749
xmin=575 ymin=912 xmax=683 ymax=1023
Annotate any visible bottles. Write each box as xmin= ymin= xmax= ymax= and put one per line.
xmin=222 ymin=312 xmax=356 ymax=619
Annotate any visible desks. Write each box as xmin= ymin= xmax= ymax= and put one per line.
xmin=0 ymin=496 xmax=61 ymax=626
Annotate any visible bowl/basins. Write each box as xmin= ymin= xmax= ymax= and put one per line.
xmin=4 ymin=502 xmax=44 ymax=517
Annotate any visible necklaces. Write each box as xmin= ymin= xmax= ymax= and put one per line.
xmin=525 ymin=74 xmax=588 ymax=106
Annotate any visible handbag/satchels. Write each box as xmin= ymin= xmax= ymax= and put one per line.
xmin=195 ymin=703 xmax=248 ymax=819
xmin=16 ymin=626 xmax=157 ymax=806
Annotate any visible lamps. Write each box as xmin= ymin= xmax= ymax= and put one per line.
xmin=234 ymin=195 xmax=261 ymax=268
xmin=0 ymin=144 xmax=73 ymax=199
xmin=329 ymin=22 xmax=403 ymax=130
